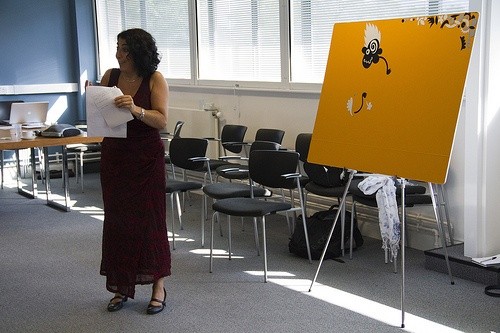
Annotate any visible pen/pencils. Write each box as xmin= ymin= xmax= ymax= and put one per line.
xmin=480 ymin=256 xmax=497 ymax=263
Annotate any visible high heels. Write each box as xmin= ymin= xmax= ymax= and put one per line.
xmin=107 ymin=296 xmax=128 ymax=312
xmin=147 ymin=287 xmax=167 ymax=314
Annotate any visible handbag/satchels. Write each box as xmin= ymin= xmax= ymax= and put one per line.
xmin=289 ymin=204 xmax=363 ymax=261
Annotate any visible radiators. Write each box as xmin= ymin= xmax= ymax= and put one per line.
xmin=167 ymin=107 xmax=222 ymax=160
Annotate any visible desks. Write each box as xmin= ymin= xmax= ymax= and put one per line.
xmin=0 ymin=126 xmax=104 ymax=212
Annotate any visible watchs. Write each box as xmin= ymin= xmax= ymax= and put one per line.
xmin=137 ymin=108 xmax=145 ymax=120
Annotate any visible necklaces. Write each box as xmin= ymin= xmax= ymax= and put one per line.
xmin=124 ymin=75 xmax=137 ymax=82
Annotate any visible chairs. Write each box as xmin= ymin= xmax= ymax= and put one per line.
xmin=73 ymin=120 xmax=102 ymax=193
xmin=165 ymin=124 xmax=454 ymax=283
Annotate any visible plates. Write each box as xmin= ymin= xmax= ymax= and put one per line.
xmin=22 ymin=136 xmax=37 ymax=140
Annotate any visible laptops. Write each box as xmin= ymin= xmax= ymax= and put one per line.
xmin=2 ymin=101 xmax=49 ymax=125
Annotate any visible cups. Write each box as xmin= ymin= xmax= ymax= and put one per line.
xmin=11 ymin=123 xmax=22 ymax=141
xmin=22 ymin=130 xmax=35 ymax=137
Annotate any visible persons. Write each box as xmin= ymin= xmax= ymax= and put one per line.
xmin=85 ymin=28 xmax=171 ymax=315
